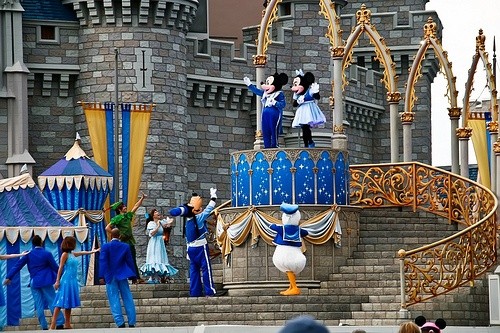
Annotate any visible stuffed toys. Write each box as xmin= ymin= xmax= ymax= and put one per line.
xmin=170 ymin=187 xmax=218 ymax=297
xmin=290 ymin=72 xmax=327 ymax=148
xmin=269 ymin=203 xmax=306 ymax=296
xmin=243 ymin=72 xmax=288 ymax=148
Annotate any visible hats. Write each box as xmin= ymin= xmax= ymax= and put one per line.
xmin=279 ymin=317 xmax=331 ymax=333
xmin=111 ymin=201 xmax=124 ymax=210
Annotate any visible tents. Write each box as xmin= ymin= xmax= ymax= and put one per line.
xmin=0 ymin=173 xmax=91 ymax=330
xmin=38 ymin=139 xmax=114 ymax=287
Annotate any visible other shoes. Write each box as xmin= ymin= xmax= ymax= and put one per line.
xmin=129 ymin=324 xmax=134 ymax=327
xmin=117 ymin=323 xmax=126 ymax=328
xmin=56 ymin=324 xmax=64 ymax=329
xmin=43 ymin=328 xmax=48 ymax=330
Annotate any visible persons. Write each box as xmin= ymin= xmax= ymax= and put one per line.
xmin=3 ymin=235 xmax=62 ymax=332
xmin=97 ymin=228 xmax=137 ymax=328
xmin=279 ymin=316 xmax=446 ymax=333
xmin=0 ymin=251 xmax=29 ymax=260
xmin=140 ymin=209 xmax=179 ymax=284
xmin=105 ymin=193 xmax=145 ymax=284
xmin=48 ymin=236 xmax=101 ymax=330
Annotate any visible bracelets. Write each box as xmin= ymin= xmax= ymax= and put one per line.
xmin=140 ymin=197 xmax=143 ymax=200
